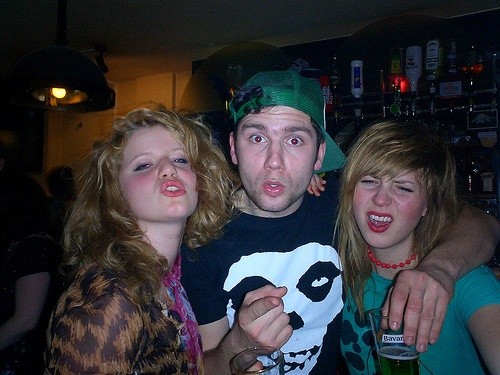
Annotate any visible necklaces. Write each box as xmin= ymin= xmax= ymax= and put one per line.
xmin=367 ymin=246 xmax=418 ymax=269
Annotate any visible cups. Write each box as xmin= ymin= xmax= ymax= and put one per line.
xmin=229 ymin=346 xmax=285 ymax=375
xmin=366 ymin=306 xmax=421 ymax=375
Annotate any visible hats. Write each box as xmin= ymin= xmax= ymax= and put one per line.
xmin=229 ymin=70 xmax=347 ymax=174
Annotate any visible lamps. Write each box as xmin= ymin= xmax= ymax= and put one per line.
xmin=4 ymin=0 xmax=116 ymax=115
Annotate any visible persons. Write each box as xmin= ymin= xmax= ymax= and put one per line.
xmin=180 ymin=68 xmax=500 ymax=375
xmin=0 ymin=141 xmax=67 ymax=375
xmin=35 ymin=164 xmax=77 ymax=243
xmin=333 ymin=116 xmax=500 ymax=375
xmin=41 ymin=110 xmax=326 ymax=375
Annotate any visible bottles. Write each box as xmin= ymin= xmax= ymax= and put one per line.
xmin=321 ymin=32 xmax=500 ymax=121
